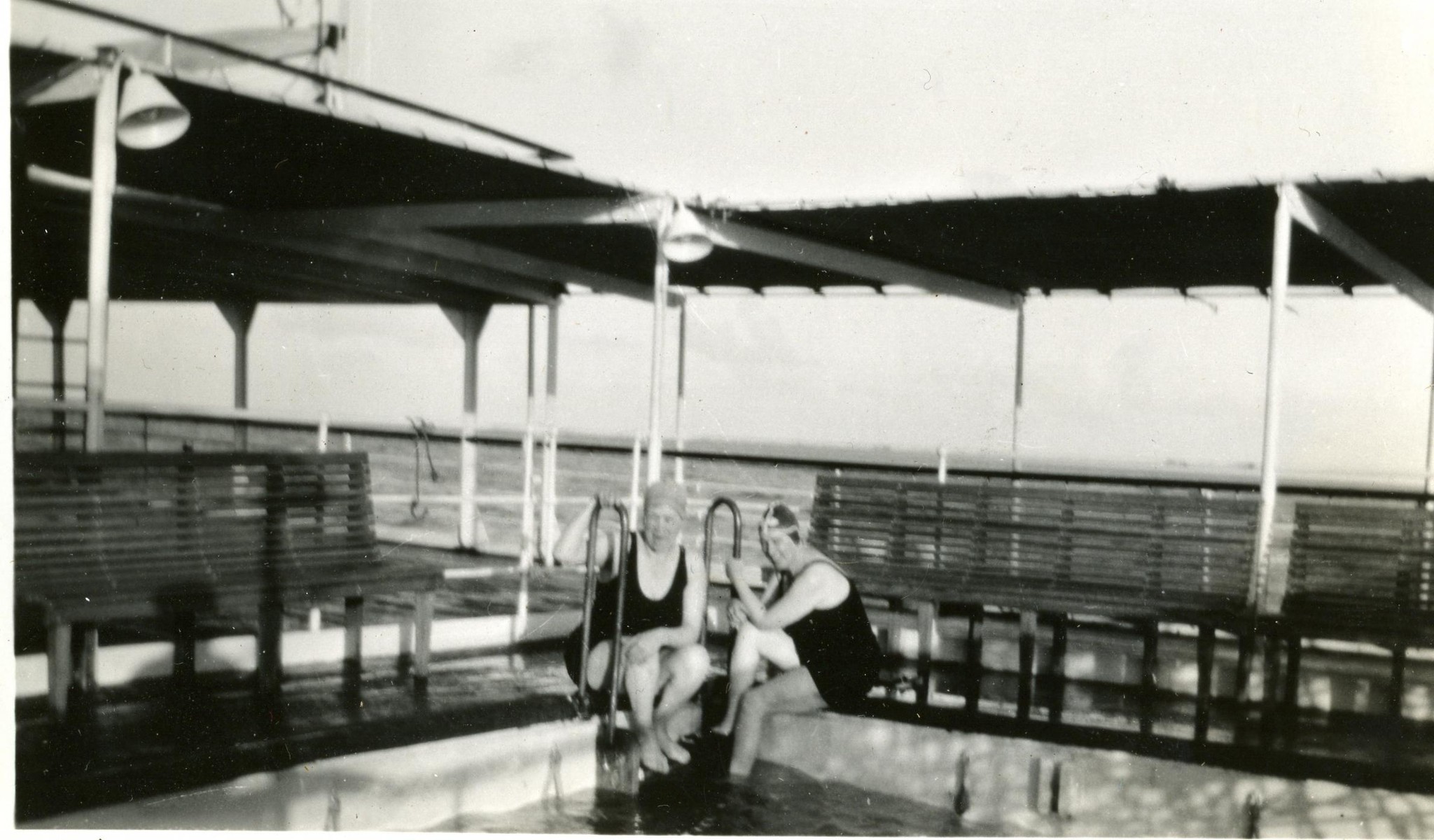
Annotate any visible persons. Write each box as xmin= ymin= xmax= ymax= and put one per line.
xmin=552 ymin=478 xmax=711 ymax=774
xmin=716 ymin=501 xmax=885 ymax=778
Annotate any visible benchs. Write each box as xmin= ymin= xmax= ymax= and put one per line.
xmin=15 ymin=451 xmax=444 ymax=762
xmin=805 ymin=473 xmax=1279 ymax=763
xmin=1257 ymin=501 xmax=1434 ymax=795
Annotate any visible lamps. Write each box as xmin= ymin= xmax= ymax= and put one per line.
xmin=663 ymin=197 xmax=713 ymax=264
xmin=113 ymin=50 xmax=192 ymax=153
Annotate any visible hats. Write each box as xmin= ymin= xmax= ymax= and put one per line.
xmin=759 ymin=505 xmax=798 ymax=538
xmin=645 ymin=481 xmax=686 ymax=518
